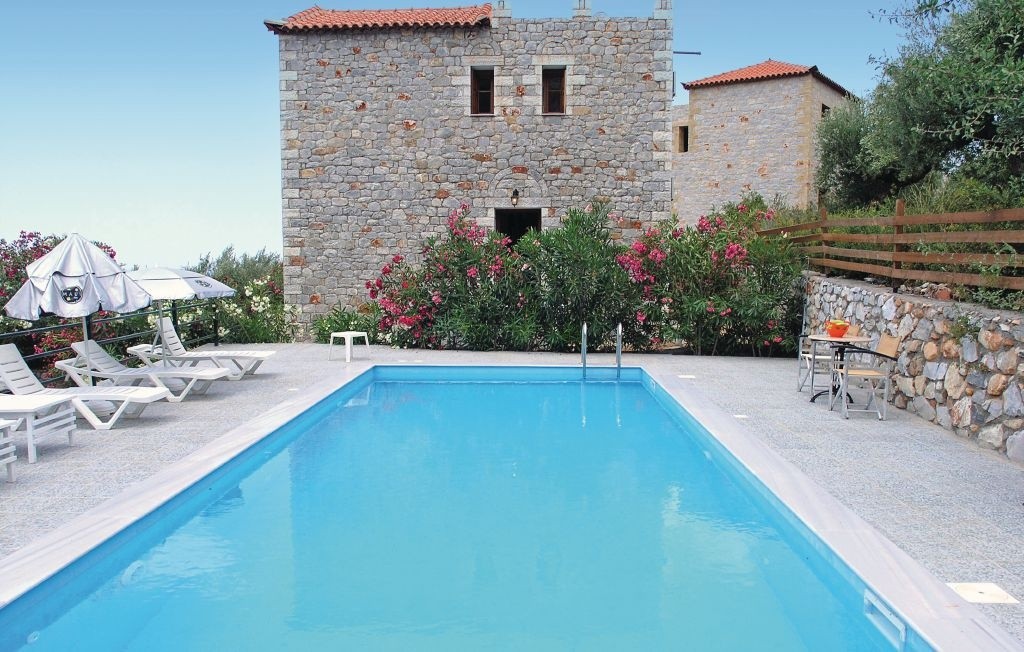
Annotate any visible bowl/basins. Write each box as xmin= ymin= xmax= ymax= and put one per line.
xmin=825 ymin=320 xmax=850 ymax=338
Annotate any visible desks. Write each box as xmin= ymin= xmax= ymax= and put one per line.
xmin=808 ymin=334 xmax=875 ymax=410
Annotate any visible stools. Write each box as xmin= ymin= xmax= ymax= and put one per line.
xmin=326 ymin=332 xmax=370 ymax=365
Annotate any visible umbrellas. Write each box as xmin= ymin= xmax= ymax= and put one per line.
xmin=1 ymin=232 xmax=154 ymax=387
xmin=127 ymin=267 xmax=237 ymax=367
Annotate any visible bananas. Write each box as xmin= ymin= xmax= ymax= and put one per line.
xmin=830 ymin=320 xmax=848 ymax=326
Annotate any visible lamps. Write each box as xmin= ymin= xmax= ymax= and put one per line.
xmin=510 ymin=189 xmax=519 ymax=207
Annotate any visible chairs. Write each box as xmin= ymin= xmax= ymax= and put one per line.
xmin=0 ymin=343 xmax=169 ymax=431
xmin=127 ymin=317 xmax=277 ymax=382
xmin=0 ymin=391 xmax=77 ymax=463
xmin=1 ymin=420 xmax=18 ymax=484
xmin=799 ymin=332 xmax=846 ymax=392
xmin=54 ymin=339 xmax=230 ymax=403
xmin=830 ymin=334 xmax=903 ymax=421
